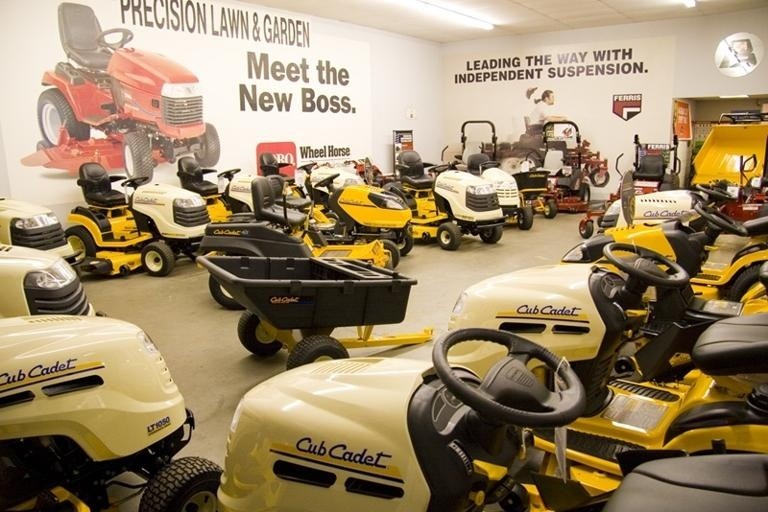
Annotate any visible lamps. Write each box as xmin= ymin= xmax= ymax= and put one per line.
xmin=385 ymin=0 xmax=494 ymax=31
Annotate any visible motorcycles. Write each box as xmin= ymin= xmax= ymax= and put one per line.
xmin=178 ymin=158 xmax=414 ymax=310
xmin=38 ymin=2 xmax=220 ymax=183
xmin=217 ymin=327 xmax=767 ymax=511
xmin=385 ymin=120 xmax=592 ymax=250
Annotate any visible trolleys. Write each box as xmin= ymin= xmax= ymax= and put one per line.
xmin=197 ymin=256 xmax=434 ymax=372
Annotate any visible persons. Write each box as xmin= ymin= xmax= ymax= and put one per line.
xmin=528 ymin=90 xmax=567 ymax=136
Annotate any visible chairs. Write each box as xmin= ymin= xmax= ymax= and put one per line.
xmin=176 ymin=156 xmax=219 ymax=196
xmin=467 ymin=153 xmax=490 ymax=175
xmin=743 ymin=215 xmax=768 ymax=236
xmin=604 ymin=449 xmax=768 ymax=512
xmin=524 ymin=117 xmax=543 ymax=138
xmin=537 ymin=150 xmax=565 ymax=175
xmin=252 ymin=177 xmax=306 ymax=227
xmin=260 ymin=152 xmax=291 ymax=178
xmin=58 ymin=2 xmax=112 ymax=69
xmin=267 ymin=175 xmax=310 ymax=209
xmin=689 ymin=311 xmax=767 ymax=378
xmin=77 ymin=162 xmax=128 ymax=206
xmin=396 ymin=151 xmax=436 ymax=188
